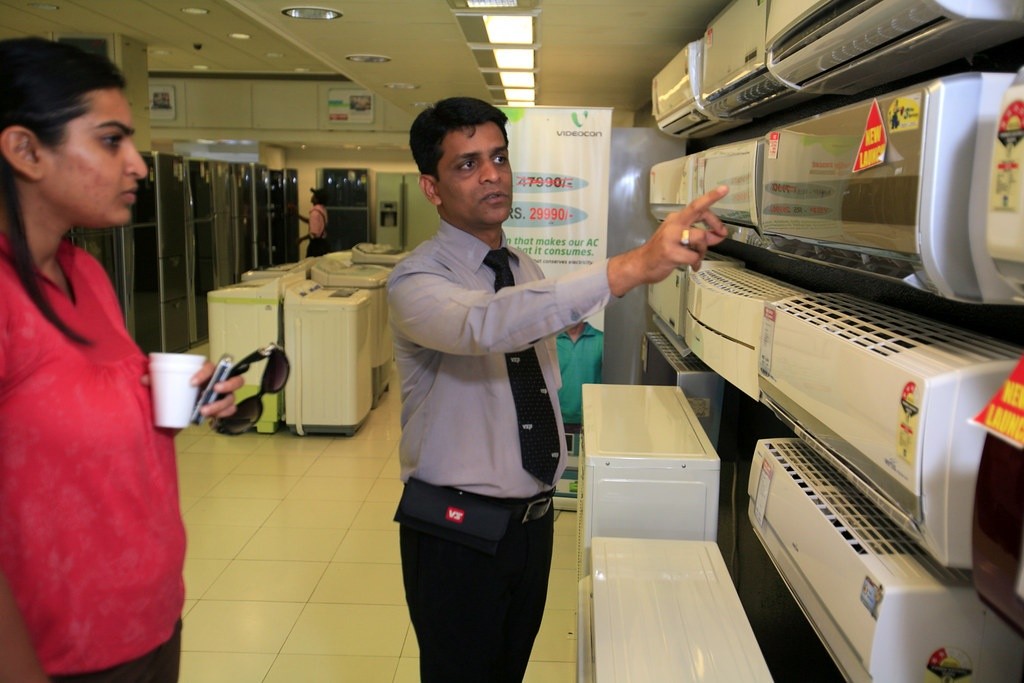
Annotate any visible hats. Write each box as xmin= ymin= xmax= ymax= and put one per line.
xmin=308 ymin=188 xmax=328 ymax=203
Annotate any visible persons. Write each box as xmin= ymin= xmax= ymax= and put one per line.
xmin=297 ymin=188 xmax=331 ymax=259
xmin=382 ymin=97 xmax=727 ymax=683
xmin=556 ymin=320 xmax=603 ymax=451
xmin=0 ymin=37 xmax=241 ymax=682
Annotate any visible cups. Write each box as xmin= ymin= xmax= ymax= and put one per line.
xmin=149 ymin=352 xmax=206 ymax=428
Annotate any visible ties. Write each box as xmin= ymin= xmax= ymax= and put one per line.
xmin=482 ymin=247 xmax=560 ymax=488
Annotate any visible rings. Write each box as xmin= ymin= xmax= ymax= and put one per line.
xmin=680 ymin=226 xmax=692 ymax=247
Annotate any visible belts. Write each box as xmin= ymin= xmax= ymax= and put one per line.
xmin=502 ymin=485 xmax=558 ymax=525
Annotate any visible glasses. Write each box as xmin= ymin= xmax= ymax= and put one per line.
xmin=207 ymin=342 xmax=290 ymax=436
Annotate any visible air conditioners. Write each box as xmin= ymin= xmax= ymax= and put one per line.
xmin=576 ymin=0 xmax=1024 ymax=683
